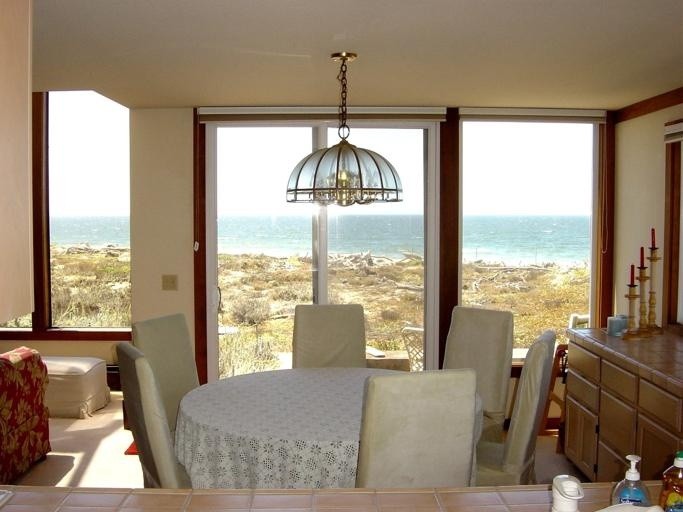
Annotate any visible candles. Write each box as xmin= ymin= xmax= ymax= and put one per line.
xmin=629 ymin=264 xmax=634 ymax=285
xmin=639 ymin=247 xmax=645 ymax=267
xmin=649 ymin=227 xmax=656 ymax=248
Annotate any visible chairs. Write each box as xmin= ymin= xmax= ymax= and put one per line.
xmin=292 ymin=304 xmax=366 ymax=370
xmin=0 ymin=345 xmax=53 ymax=484
xmin=115 ymin=341 xmax=192 ymax=490
xmin=567 ymin=314 xmax=588 ymax=345
xmin=355 ymin=369 xmax=476 ymax=489
xmin=476 ymin=330 xmax=555 ymax=487
xmin=401 ymin=327 xmax=425 ymax=370
xmin=131 ymin=313 xmax=202 ymax=433
xmin=441 ymin=306 xmax=514 ymax=440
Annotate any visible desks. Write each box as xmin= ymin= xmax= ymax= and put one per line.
xmin=511 ymin=348 xmax=530 ymax=364
xmin=219 ymin=326 xmax=238 ymax=337
xmin=365 ymin=348 xmax=409 ymax=370
xmin=177 ymin=369 xmax=484 ymax=489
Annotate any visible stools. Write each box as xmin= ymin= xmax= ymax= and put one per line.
xmin=40 ymin=355 xmax=112 ymax=420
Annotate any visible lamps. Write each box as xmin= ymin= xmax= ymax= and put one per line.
xmin=286 ymin=53 xmax=406 ymax=206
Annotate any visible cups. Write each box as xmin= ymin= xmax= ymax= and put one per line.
xmin=606 ymin=313 xmax=629 ymax=336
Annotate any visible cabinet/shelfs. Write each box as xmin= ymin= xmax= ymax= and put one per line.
xmin=557 ymin=325 xmax=682 ymax=482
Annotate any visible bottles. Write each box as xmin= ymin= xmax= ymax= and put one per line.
xmin=658 ymin=450 xmax=683 ymax=512
